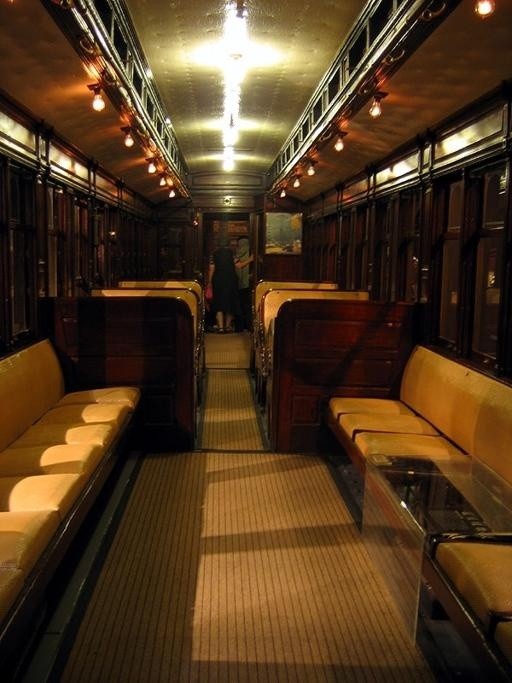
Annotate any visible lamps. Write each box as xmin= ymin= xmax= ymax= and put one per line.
xmin=276 ymin=91 xmax=388 ymax=198
xmin=88 ymin=83 xmax=180 ymax=200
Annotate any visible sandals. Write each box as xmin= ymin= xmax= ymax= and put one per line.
xmin=217 ymin=327 xmax=224 ymax=332
xmin=225 ymin=327 xmax=233 ymax=333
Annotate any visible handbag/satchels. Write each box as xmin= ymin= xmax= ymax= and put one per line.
xmin=206 ymin=284 xmax=213 ymax=299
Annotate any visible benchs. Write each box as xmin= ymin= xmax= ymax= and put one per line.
xmin=30 ymin=272 xmax=207 ymax=450
xmin=251 ymin=275 xmax=427 ymax=454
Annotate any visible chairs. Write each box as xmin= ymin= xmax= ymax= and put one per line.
xmin=0 ymin=337 xmax=142 ymax=614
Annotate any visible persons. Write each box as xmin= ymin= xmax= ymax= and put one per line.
xmin=206 ymin=237 xmax=254 ymax=334
